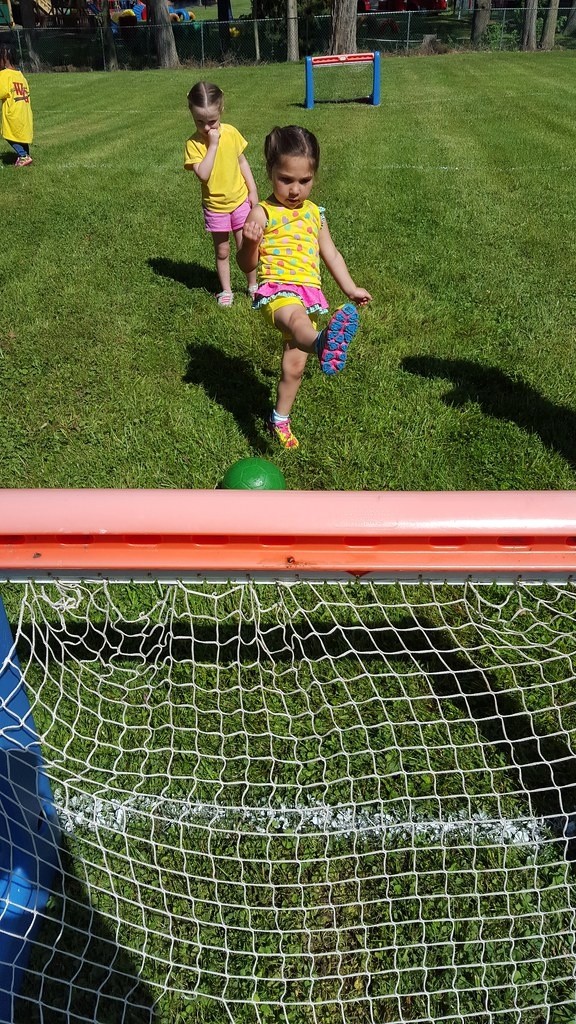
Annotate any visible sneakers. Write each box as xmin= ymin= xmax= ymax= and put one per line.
xmin=14 ymin=154 xmax=33 ymax=167
xmin=266 ymin=413 xmax=299 ymax=452
xmin=248 ymin=283 xmax=258 ymax=298
xmin=215 ymin=290 xmax=234 ymax=307
xmin=317 ymin=303 xmax=359 ymax=376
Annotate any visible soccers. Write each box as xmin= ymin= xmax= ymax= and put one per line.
xmin=228 ymin=459 xmax=285 ymax=489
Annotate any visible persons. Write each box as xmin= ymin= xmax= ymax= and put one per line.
xmin=0 ymin=43 xmax=34 ymax=167
xmin=185 ymin=80 xmax=259 ymax=307
xmin=237 ymin=126 xmax=375 ymax=450
